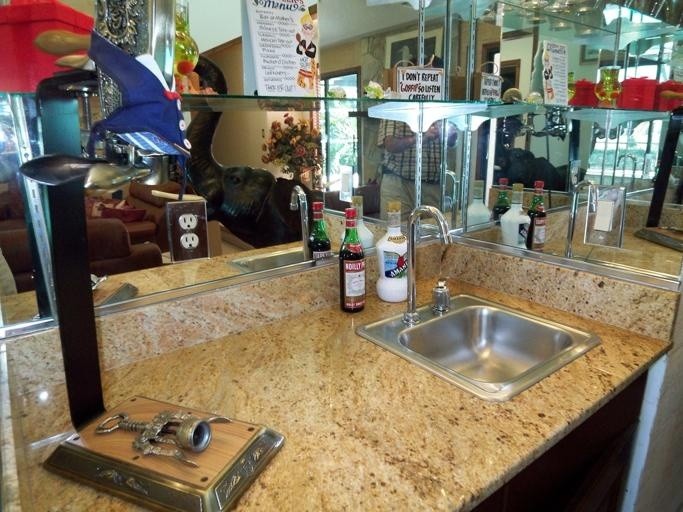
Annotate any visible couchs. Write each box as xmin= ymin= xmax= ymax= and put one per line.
xmin=1 ymin=180 xmax=186 ymax=295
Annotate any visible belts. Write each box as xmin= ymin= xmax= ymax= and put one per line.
xmin=387 ymin=170 xmax=440 ymax=185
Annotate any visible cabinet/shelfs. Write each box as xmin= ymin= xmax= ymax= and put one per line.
xmin=470 ymin=368 xmax=647 ymax=512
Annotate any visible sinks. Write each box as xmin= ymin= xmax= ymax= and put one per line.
xmin=355 ymin=293 xmax=601 ymax=402
xmin=228 ymin=247 xmax=304 ymax=272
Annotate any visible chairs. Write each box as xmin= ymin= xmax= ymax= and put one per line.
xmin=476 ymin=145 xmax=586 ymax=192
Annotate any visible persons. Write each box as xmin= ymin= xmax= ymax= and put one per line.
xmin=375 ymin=56 xmax=458 ymax=221
xmin=544 ymin=53 xmax=554 ymax=99
xmin=296 ymin=24 xmax=318 ymax=88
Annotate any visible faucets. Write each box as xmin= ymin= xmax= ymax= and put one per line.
xmin=565 ymin=180 xmax=597 ymax=258
xmin=289 ymin=183 xmax=311 ymax=261
xmin=406 ymin=205 xmax=452 ymax=313
xmin=616 ymin=153 xmax=637 ymax=192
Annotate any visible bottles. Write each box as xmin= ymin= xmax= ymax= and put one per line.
xmin=306 ymin=202 xmax=332 ymax=258
xmin=568 ymin=72 xmax=576 ymax=102
xmin=339 ymin=208 xmax=366 ymax=312
xmin=173 ymin=0 xmax=199 ymax=76
xmin=342 ymin=195 xmax=373 ymax=248
xmin=493 ymin=177 xmax=547 ymax=250
xmin=377 ymin=200 xmax=408 ymax=303
xmin=594 ymin=66 xmax=623 ymax=109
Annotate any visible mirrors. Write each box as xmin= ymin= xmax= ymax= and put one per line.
xmin=446 ymin=1 xmax=683 ymax=296
xmin=2 ymin=2 xmax=443 ymax=336
